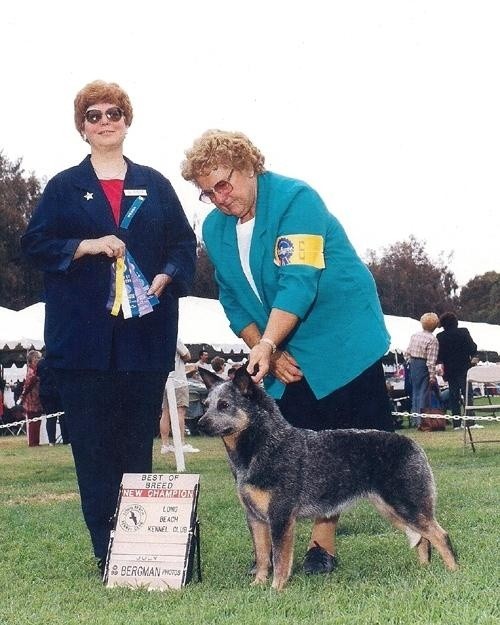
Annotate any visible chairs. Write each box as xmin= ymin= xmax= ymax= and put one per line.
xmin=461 ymin=363 xmax=500 ymax=451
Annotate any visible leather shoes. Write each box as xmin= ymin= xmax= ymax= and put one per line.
xmin=247 ymin=546 xmax=274 ymax=580
xmin=300 ymin=543 xmax=339 ymax=577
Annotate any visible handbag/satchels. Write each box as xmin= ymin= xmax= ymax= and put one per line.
xmin=416 ymin=406 xmax=448 ymax=433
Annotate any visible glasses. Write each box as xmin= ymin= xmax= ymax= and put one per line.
xmin=194 ymin=167 xmax=237 ymax=205
xmin=81 ymin=106 xmax=126 ymax=126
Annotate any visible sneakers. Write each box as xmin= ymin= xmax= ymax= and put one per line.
xmin=181 ymin=442 xmax=200 ymax=455
xmin=464 ymin=423 xmax=486 ymax=430
xmin=160 ymin=444 xmax=175 ymax=454
xmin=452 ymin=424 xmax=463 ymax=432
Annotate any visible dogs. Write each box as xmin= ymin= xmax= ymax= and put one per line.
xmin=191 ymin=363 xmax=461 ymax=593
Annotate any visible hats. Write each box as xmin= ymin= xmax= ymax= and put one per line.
xmin=184 ymin=364 xmax=198 ymax=376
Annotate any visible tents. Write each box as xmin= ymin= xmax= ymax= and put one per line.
xmin=0 ymin=294 xmax=500 ymax=363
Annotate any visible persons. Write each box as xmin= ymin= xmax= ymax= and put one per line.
xmin=0 ymin=379 xmax=7 ymax=437
xmin=19 ymin=77 xmax=199 ymax=577
xmin=404 ymin=313 xmax=437 ymax=428
xmin=433 ymin=313 xmax=484 ymax=430
xmin=0 ymin=340 xmax=458 ymax=453
xmin=177 ymin=128 xmax=397 ymax=576
